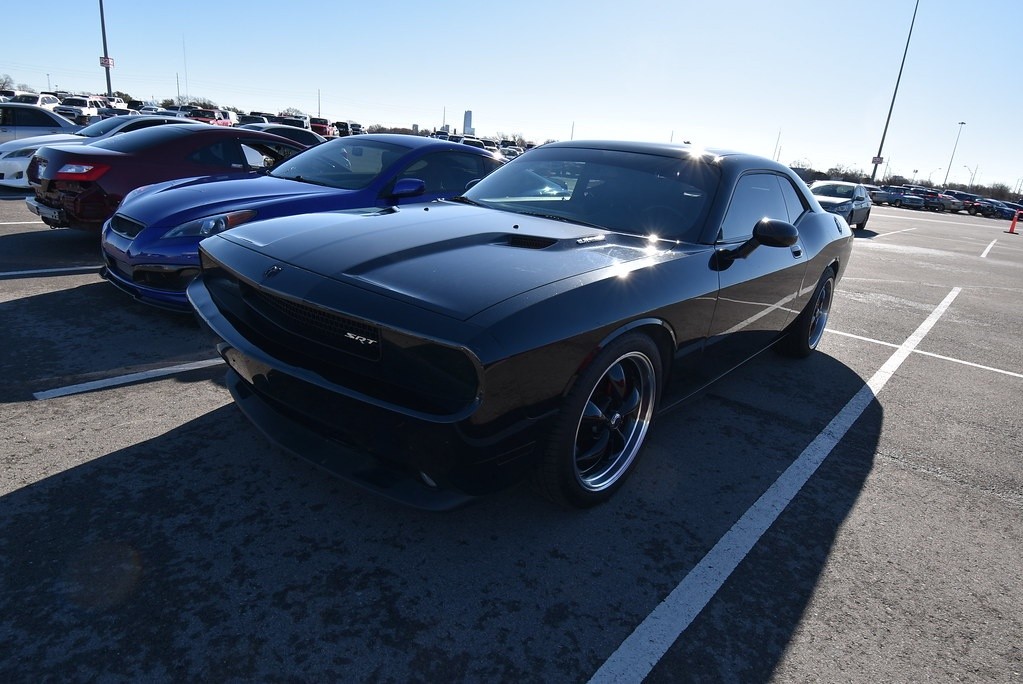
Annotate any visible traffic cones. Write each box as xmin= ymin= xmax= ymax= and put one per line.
xmin=1004 ymin=209 xmax=1020 ymax=235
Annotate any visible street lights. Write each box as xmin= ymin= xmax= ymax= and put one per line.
xmin=928 ymin=168 xmax=942 ymax=181
xmin=964 ymin=166 xmax=974 ymax=188
xmin=945 ymin=121 xmax=966 ymax=184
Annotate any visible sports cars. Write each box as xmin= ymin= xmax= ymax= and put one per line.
xmin=99 ymin=132 xmax=574 ymax=320
xmin=183 ymin=138 xmax=856 ymax=510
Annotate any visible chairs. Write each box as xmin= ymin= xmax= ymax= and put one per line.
xmin=657 ymin=194 xmax=717 ymax=236
xmin=412 ymin=158 xmax=461 ymax=193
xmin=580 ymin=178 xmax=646 ymax=223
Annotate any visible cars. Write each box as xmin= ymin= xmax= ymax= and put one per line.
xmin=0 ymin=114 xmax=204 ymax=190
xmin=25 ymin=123 xmax=363 ymax=237
xmin=428 ymin=131 xmax=585 ymax=177
xmin=809 ymin=180 xmax=872 ymax=230
xmin=862 ymin=183 xmax=1023 ymax=221
xmin=240 ymin=121 xmax=351 ymax=170
xmin=0 ymin=90 xmax=371 ymax=144
